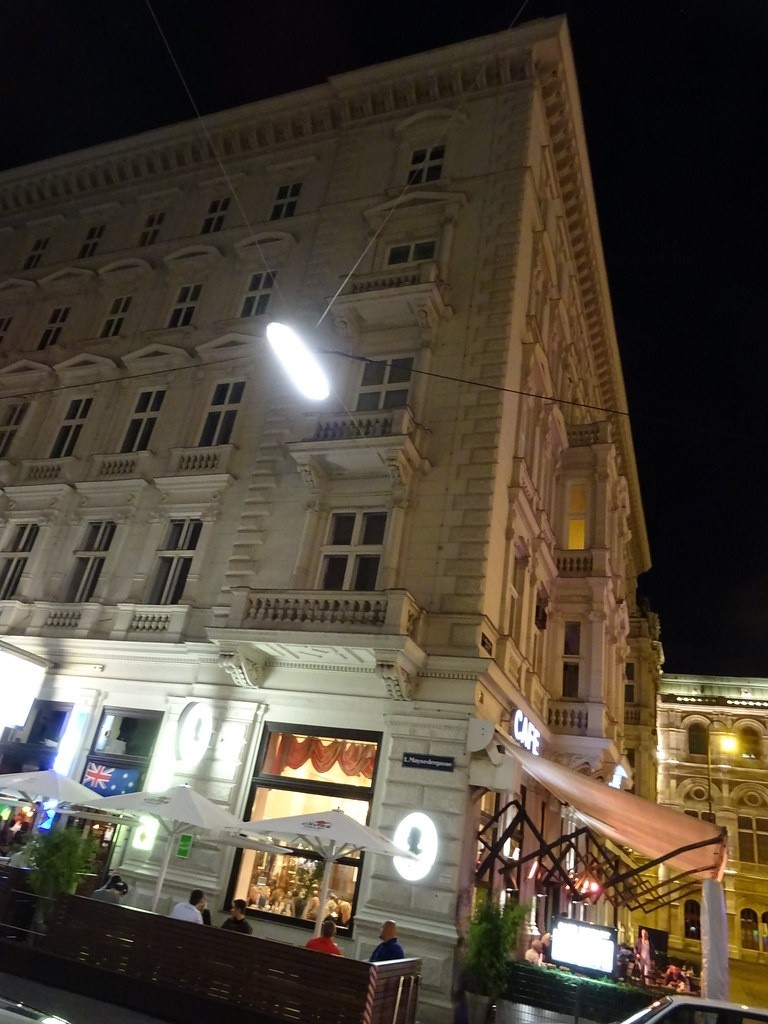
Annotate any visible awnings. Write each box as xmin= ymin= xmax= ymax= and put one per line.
xmin=498 ymin=735 xmax=729 ymax=913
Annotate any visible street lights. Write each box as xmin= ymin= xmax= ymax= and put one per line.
xmin=707 ymin=719 xmax=740 ymax=823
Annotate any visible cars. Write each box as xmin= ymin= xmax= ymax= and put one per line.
xmin=612 ymin=993 xmax=768 ymax=1024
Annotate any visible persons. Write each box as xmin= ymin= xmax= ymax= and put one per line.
xmin=541 ymin=933 xmax=570 ymax=971
xmin=0 ymin=819 xmax=17 ymax=847
xmin=368 ymin=920 xmax=404 ymax=962
xmin=197 ymin=896 xmax=211 ymax=926
xmin=656 ymin=954 xmax=690 ymax=993
xmin=92 ymin=875 xmax=128 ymax=904
xmin=305 ymin=921 xmax=344 ymax=956
xmin=524 ymin=939 xmax=547 ymax=968
xmin=221 ymin=899 xmax=254 ymax=935
xmin=168 ymin=890 xmax=204 ymax=925
xmin=618 ymin=942 xmax=635 ymax=979
xmin=633 ymin=928 xmax=656 ymax=986
xmin=4 ymin=821 xmax=29 ymax=856
xmin=246 ymin=884 xmax=351 ymax=927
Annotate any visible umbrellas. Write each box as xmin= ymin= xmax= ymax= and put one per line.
xmin=224 ymin=806 xmax=419 ymax=938
xmin=70 ymin=782 xmax=272 ymax=912
xmin=0 ymin=769 xmax=142 ymax=850
